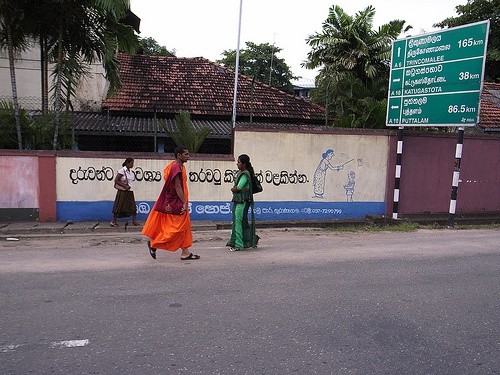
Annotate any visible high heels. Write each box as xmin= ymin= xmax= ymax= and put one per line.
xmin=230 ymin=247 xmax=240 ymax=251
xmin=254 ymin=235 xmax=261 ymax=248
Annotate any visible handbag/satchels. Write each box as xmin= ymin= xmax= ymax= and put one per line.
xmin=154 ymin=164 xmax=185 ymax=215
xmin=114 ymin=169 xmax=130 ymax=190
xmin=252 ymin=177 xmax=263 ymax=194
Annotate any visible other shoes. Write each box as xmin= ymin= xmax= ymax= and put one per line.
xmin=132 ymin=223 xmax=142 ymax=226
xmin=110 ymin=222 xmax=119 ymax=227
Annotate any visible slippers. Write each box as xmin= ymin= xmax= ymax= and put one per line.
xmin=181 ymin=253 xmax=200 ymax=260
xmin=147 ymin=240 xmax=156 ymax=259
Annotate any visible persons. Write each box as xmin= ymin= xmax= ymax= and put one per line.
xmin=142 ymin=145 xmax=200 ymax=260
xmin=110 ymin=158 xmax=140 ymax=226
xmin=227 ymin=154 xmax=261 ymax=251
